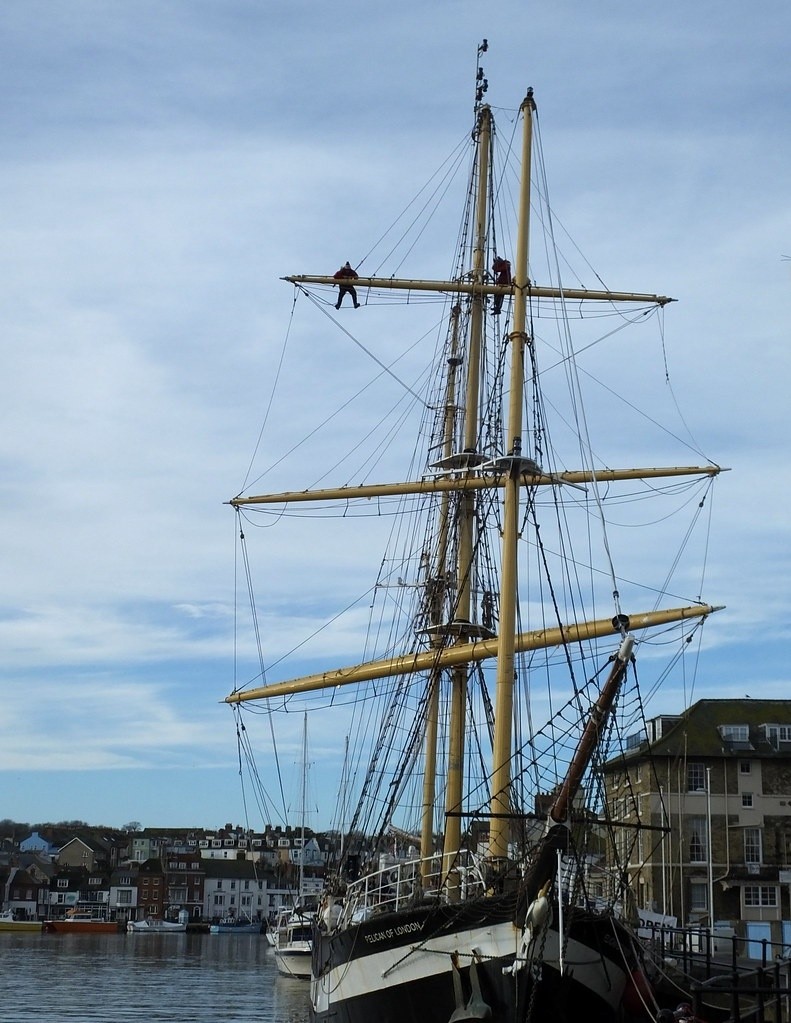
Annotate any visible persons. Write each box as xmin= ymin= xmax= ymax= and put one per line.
xmin=490 ymin=256 xmax=512 ymax=315
xmin=334 ymin=261 xmax=360 ymax=310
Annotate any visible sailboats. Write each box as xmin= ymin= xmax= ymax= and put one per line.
xmin=218 ymin=35 xmax=734 ymax=1023
xmin=263 ymin=703 xmax=337 ymax=979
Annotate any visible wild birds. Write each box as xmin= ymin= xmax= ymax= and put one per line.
xmin=744 ymin=694 xmax=751 ymax=699
xmin=509 ymin=880 xmax=554 ymax=981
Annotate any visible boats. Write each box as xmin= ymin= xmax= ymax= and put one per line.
xmin=209 ymin=921 xmax=261 ymax=935
xmin=126 ymin=915 xmax=187 ymax=933
